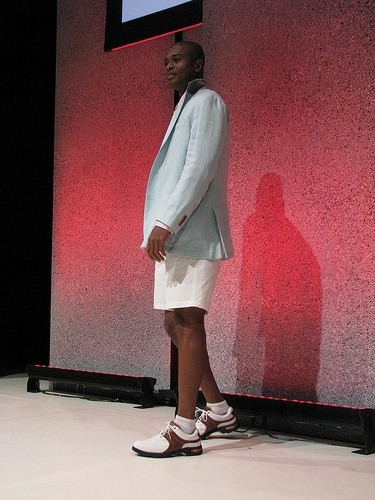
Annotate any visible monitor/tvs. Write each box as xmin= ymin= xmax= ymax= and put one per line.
xmin=104 ymin=0 xmax=202 ymax=53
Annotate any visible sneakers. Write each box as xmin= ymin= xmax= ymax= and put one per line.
xmin=195 ymin=405 xmax=238 ymax=439
xmin=131 ymin=422 xmax=203 ymax=456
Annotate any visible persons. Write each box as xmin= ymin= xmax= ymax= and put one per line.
xmin=131 ymin=40 xmax=239 ymax=458
